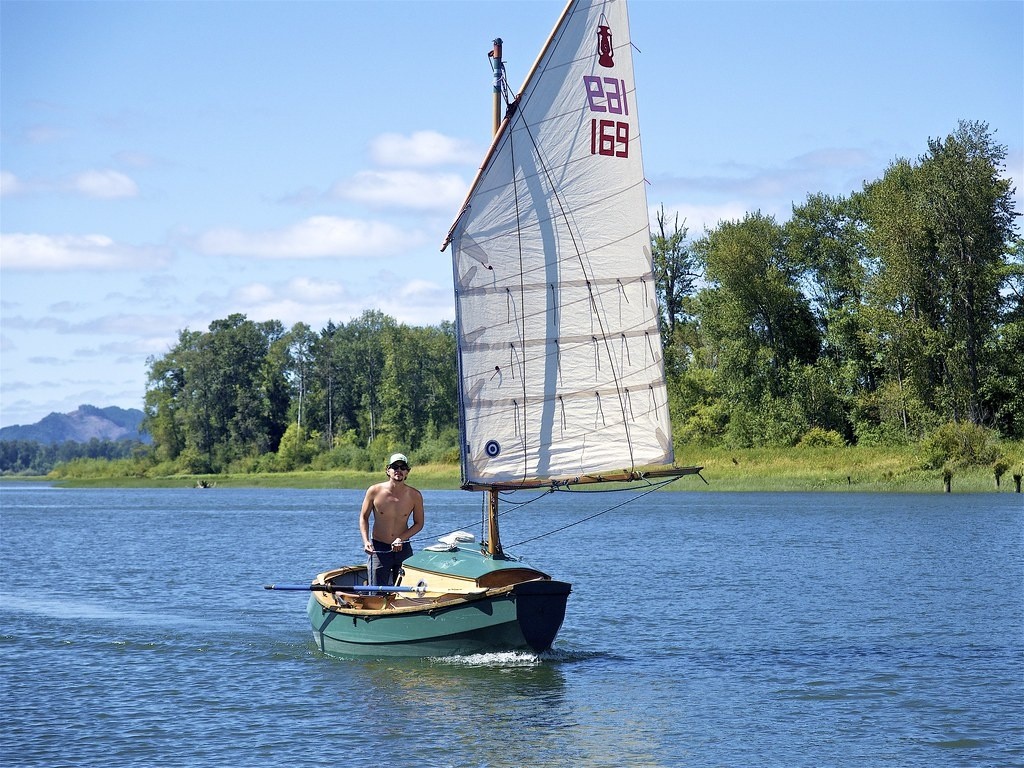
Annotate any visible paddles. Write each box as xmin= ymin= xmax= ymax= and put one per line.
xmin=263 ymin=583 xmax=490 ymax=596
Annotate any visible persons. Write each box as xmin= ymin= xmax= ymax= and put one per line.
xmin=360 ymin=454 xmax=424 ymax=595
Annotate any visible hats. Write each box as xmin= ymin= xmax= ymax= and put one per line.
xmin=389 ymin=454 xmax=408 ymax=465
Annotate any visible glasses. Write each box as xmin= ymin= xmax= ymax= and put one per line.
xmin=388 ymin=464 xmax=409 ymax=471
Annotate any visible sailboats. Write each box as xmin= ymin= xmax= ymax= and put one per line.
xmin=305 ymin=0 xmax=704 ymax=656
